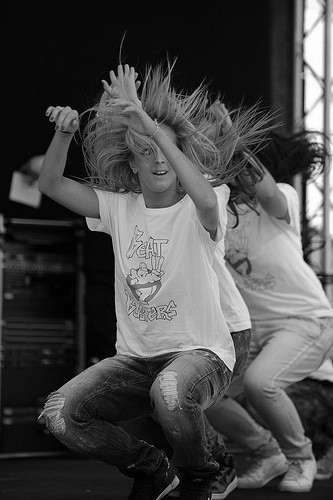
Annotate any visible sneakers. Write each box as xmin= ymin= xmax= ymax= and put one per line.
xmin=180 ymin=453 xmax=238 ymax=500
xmin=277 ymin=452 xmax=317 ymax=493
xmin=128 ymin=450 xmax=180 ymax=500
xmin=237 ymin=446 xmax=290 ymax=489
xmin=314 ymin=445 xmax=333 ymax=480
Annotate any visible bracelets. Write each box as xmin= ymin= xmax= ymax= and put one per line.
xmin=59 ymin=130 xmax=73 ymax=134
xmin=147 ymin=118 xmax=159 ymax=138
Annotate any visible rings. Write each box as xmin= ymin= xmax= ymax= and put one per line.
xmin=55 ymin=106 xmax=62 ymax=112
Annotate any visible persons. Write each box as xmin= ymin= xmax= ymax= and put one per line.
xmin=38 ymin=52 xmax=286 ymax=500
xmin=199 ymin=99 xmax=333 ymax=492
xmin=95 ymin=65 xmax=267 ymax=499
